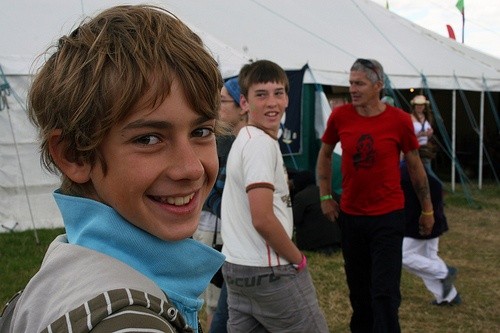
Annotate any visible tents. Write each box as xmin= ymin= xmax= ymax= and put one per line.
xmin=0 ymin=0 xmax=500 ymax=233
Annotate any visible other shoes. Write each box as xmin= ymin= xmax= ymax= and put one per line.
xmin=432 ymin=265 xmax=461 ymax=304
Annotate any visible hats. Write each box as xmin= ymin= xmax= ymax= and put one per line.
xmin=410 ymin=95 xmax=430 ymax=105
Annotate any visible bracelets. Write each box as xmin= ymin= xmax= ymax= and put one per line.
xmin=320 ymin=195 xmax=332 ymax=201
xmin=422 ymin=211 xmax=433 ymax=216
xmin=293 ymin=255 xmax=307 ymax=270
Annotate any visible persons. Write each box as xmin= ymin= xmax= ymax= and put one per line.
xmin=188 ymin=78 xmax=249 ymax=333
xmin=317 ymin=59 xmax=435 ymax=333
xmin=10 ymin=4 xmax=223 ymax=333
xmin=400 ymin=144 xmax=462 ymax=306
xmin=410 ymin=95 xmax=433 ymax=145
xmin=221 ymin=60 xmax=328 ymax=333
